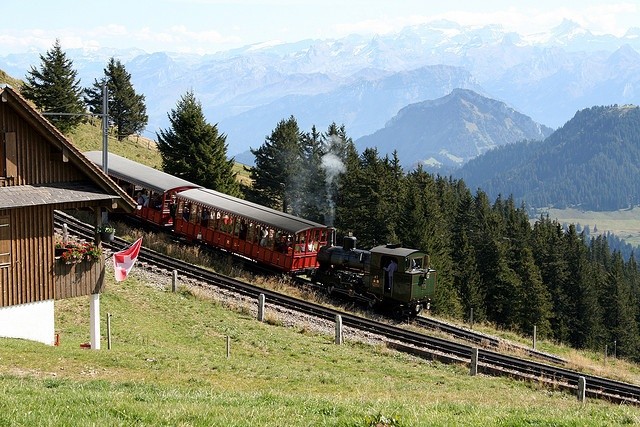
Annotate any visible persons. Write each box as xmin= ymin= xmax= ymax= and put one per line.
xmin=181 ymin=200 xmax=294 ymax=254
xmin=137 ymin=188 xmax=161 ymax=208
xmin=383 ymin=258 xmax=397 ymax=289
xmin=168 ymin=195 xmax=177 ymax=223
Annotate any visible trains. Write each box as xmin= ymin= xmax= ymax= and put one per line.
xmin=85 ymin=149 xmax=437 ymax=322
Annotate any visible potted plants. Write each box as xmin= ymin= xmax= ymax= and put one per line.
xmin=100 ymin=227 xmax=116 ymax=242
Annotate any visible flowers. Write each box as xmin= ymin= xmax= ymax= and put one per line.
xmin=60 ymin=246 xmax=102 ymax=266
xmin=54 ymin=240 xmax=93 ymax=250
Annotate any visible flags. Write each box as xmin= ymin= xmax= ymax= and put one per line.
xmin=113 ymin=239 xmax=143 ymax=281
